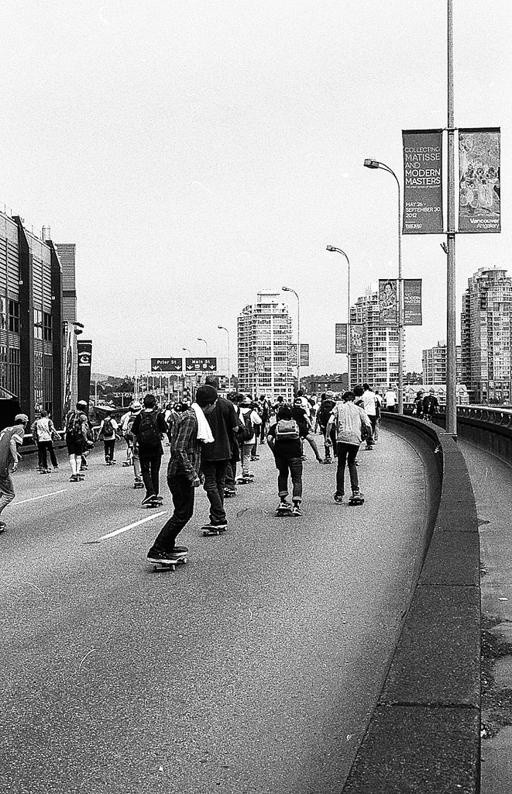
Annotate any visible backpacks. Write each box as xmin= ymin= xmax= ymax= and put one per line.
xmin=138 ymin=410 xmax=163 ymax=450
xmin=235 ymin=410 xmax=254 ymax=442
xmin=68 ymin=409 xmax=85 ymax=437
xmin=101 ymin=416 xmax=114 ymax=438
xmin=320 ymin=399 xmax=336 ymax=429
xmin=124 ymin=413 xmax=138 ymax=442
xmin=275 ymin=419 xmax=300 ymax=441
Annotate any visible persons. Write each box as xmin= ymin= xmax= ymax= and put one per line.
xmin=0 ymin=412 xmax=30 ymax=533
xmin=286 ymin=344 xmax=297 ymax=366
xmin=199 ymin=374 xmax=242 ymax=535
xmin=34 ymin=410 xmax=55 ymax=469
xmin=375 ymin=386 xmax=440 ymax=423
xmin=64 ymin=399 xmax=94 ymax=481
xmin=29 ymin=410 xmax=62 ymax=471
xmin=145 ymin=385 xmax=218 ymax=565
xmin=378 ymin=279 xmax=400 ymax=327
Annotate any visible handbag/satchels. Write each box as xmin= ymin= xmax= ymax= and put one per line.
xmin=359 ymin=422 xmax=372 ymax=441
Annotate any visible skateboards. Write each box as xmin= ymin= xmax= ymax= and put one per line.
xmin=150 ymin=555 xmax=188 ymax=571
xmin=134 ymin=481 xmax=144 ymax=489
xmin=224 ymin=487 xmax=237 ymax=498
xmin=200 ymin=524 xmax=227 ymax=536
xmin=348 ymin=492 xmax=365 ymax=505
xmin=237 ymin=477 xmax=254 ymax=484
xmin=149 ymin=498 xmax=162 ymax=507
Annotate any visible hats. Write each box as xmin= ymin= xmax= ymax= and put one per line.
xmin=40 ymin=410 xmax=48 ymax=416
xmin=77 ymin=399 xmax=88 ymax=408
xmin=294 ymin=398 xmax=303 ymax=406
xmin=14 ymin=413 xmax=30 ymax=423
xmin=240 ymin=396 xmax=253 ymax=405
xmin=325 ymin=391 xmax=334 ymax=399
xmin=130 ymin=401 xmax=142 ymax=410
xmin=174 ymin=402 xmax=182 ymax=411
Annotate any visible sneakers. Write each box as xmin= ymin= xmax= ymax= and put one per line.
xmin=105 ymin=454 xmax=113 ymax=462
xmin=147 ymin=546 xmax=187 ymax=563
xmin=134 ymin=477 xmax=143 ymax=483
xmin=251 ymin=454 xmax=259 ymax=457
xmin=352 ymin=488 xmax=361 ymax=496
xmin=141 ymin=492 xmax=163 ymax=505
xmin=334 ymin=493 xmax=343 ymax=503
xmin=258 ymin=434 xmax=269 ymax=445
xmin=278 ymin=501 xmax=292 ymax=508
xmin=36 ymin=463 xmax=60 ymax=475
xmin=201 ymin=518 xmax=229 ymax=529
xmin=292 ymin=505 xmax=305 ymax=517
xmin=70 ymin=474 xmax=85 ymax=479
xmin=224 ymin=484 xmax=238 ymax=492
xmin=242 ymin=468 xmax=255 ymax=477
xmin=316 ymin=454 xmax=332 ymax=465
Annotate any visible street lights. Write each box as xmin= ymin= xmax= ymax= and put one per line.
xmin=180 ymin=346 xmax=193 ymax=355
xmin=196 ymin=337 xmax=209 ymax=356
xmin=362 ymin=157 xmax=406 ymax=416
xmin=325 ymin=243 xmax=352 ymax=395
xmin=281 ymin=284 xmax=302 ymax=395
xmin=217 ymin=325 xmax=231 ymax=376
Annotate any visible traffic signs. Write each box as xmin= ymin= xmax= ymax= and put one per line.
xmin=151 ymin=358 xmax=182 ymax=372
xmin=185 ymin=357 xmax=218 ymax=372
